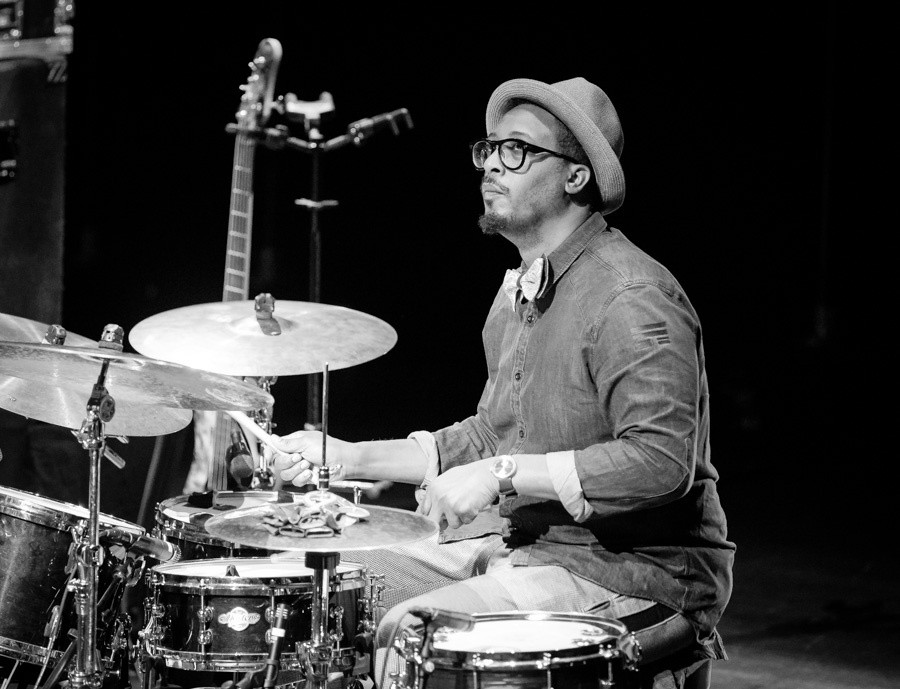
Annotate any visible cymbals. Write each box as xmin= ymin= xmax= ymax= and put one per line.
xmin=0 ymin=313 xmax=193 ymax=436
xmin=128 ymin=292 xmax=398 ymax=377
xmin=0 ymin=323 xmax=274 ymax=411
xmin=204 ymin=500 xmax=441 ymax=553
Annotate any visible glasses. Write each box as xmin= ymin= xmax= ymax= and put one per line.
xmin=469 ymin=139 xmax=581 ymax=170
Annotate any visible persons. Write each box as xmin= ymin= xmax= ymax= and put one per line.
xmin=262 ymin=77 xmax=738 ymax=689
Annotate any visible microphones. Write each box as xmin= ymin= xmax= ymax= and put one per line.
xmin=229 ymin=429 xmax=253 ymax=480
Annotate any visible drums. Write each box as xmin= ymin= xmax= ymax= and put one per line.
xmin=151 ymin=557 xmax=370 ymax=672
xmin=0 ymin=485 xmax=146 ymax=676
xmin=153 ymin=489 xmax=308 ymax=562
xmin=406 ymin=609 xmax=639 ymax=689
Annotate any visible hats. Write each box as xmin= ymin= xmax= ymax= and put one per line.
xmin=486 ymin=77 xmax=625 ymax=216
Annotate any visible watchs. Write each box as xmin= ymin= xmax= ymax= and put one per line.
xmin=489 ymin=454 xmax=517 ymax=496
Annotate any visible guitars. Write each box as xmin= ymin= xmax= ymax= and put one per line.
xmin=211 ymin=37 xmax=283 ymax=495
